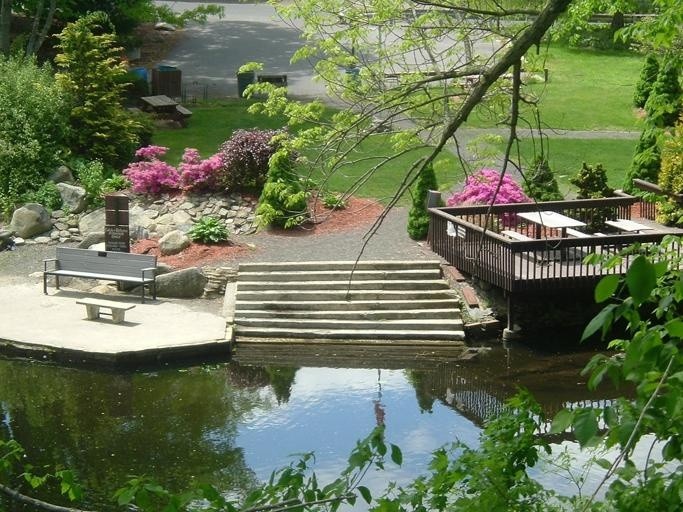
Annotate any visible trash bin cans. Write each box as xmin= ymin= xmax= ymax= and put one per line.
xmin=236 ymin=71 xmax=255 ymax=99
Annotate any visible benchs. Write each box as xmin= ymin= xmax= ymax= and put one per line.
xmin=557 ymin=227 xmax=600 ymax=254
xmin=74 ymin=297 xmax=135 ymax=323
xmin=633 ymin=230 xmax=645 ymax=234
xmin=173 ymin=103 xmax=193 ymax=128
xmin=501 ymin=229 xmax=543 ymax=263
xmin=42 ymin=245 xmax=157 ymax=304
xmin=593 ymin=231 xmax=606 ymax=240
xmin=128 ymin=107 xmax=142 ymax=116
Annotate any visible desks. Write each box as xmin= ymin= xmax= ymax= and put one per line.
xmin=515 ymin=210 xmax=586 ymax=249
xmin=139 ymin=94 xmax=178 ymax=127
xmin=604 ymin=218 xmax=653 ymax=246
